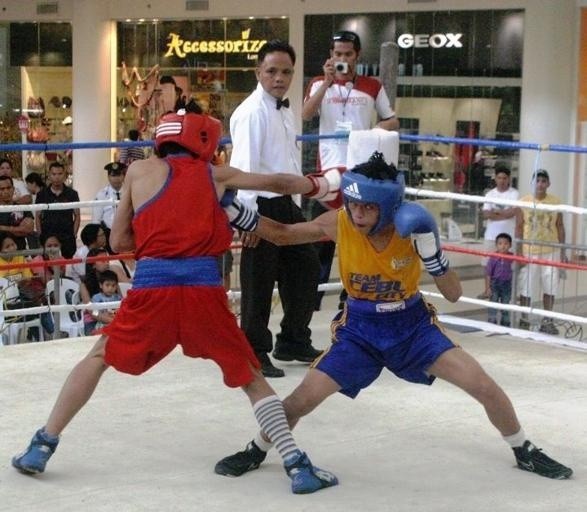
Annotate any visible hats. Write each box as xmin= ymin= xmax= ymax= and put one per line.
xmin=104 ymin=161 xmax=128 ymax=174
xmin=532 ymin=169 xmax=549 ymax=179
xmin=52 ymin=96 xmax=72 ymax=108
xmin=64 ymin=116 xmax=73 ymax=125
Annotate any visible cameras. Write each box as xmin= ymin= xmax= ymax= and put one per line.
xmin=333 ymin=62 xmax=348 ymax=74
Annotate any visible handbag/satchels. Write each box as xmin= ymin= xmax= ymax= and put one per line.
xmin=24 ymin=97 xmax=50 ymax=171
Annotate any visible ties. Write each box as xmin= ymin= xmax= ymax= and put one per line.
xmin=116 ymin=192 xmax=120 ymax=207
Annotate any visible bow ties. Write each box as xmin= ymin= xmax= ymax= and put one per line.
xmin=275 ymin=96 xmax=291 ymax=111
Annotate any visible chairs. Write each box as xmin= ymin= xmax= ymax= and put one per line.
xmin=115 ymin=281 xmax=133 ymax=294
xmin=0 ymin=274 xmax=48 ymax=345
xmin=44 ymin=277 xmax=89 ymax=337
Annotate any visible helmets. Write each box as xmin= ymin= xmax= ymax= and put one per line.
xmin=153 ymin=108 xmax=224 ymax=164
xmin=339 ymin=159 xmax=406 ymax=238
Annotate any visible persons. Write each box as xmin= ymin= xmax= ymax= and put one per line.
xmin=477 ymin=165 xmax=520 ymax=299
xmin=228 ymin=41 xmax=323 ymax=377
xmin=13 ymin=108 xmax=341 ymax=495
xmin=303 ymin=30 xmax=401 ymax=312
xmin=515 ymin=168 xmax=567 ymax=335
xmin=214 ymin=152 xmax=574 ymax=481
xmin=483 ymin=232 xmax=515 ymax=327
xmin=0 ymin=130 xmax=145 ymax=346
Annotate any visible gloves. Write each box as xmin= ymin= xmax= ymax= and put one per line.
xmin=302 ymin=164 xmax=348 ymax=211
xmin=391 ymin=200 xmax=440 ymax=262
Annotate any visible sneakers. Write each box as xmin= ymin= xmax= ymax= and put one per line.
xmin=540 ymin=316 xmax=559 ymax=335
xmin=519 ymin=317 xmax=530 ymax=330
xmin=11 ymin=427 xmax=59 ymax=475
xmin=213 ymin=440 xmax=267 ymax=477
xmin=512 ymin=439 xmax=573 ymax=479
xmin=273 ymin=334 xmax=324 ymax=362
xmin=282 ymin=452 xmax=339 ymax=493
xmin=488 ymin=318 xmax=511 ymax=326
xmin=256 ymin=350 xmax=284 ymax=377
